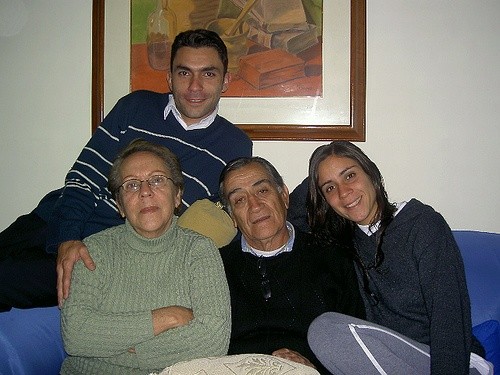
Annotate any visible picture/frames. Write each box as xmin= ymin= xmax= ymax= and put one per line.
xmin=93 ymin=0 xmax=367 ymax=142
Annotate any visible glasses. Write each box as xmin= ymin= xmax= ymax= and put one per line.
xmin=256 ymin=255 xmax=271 ymax=302
xmin=115 ymin=175 xmax=178 ymax=201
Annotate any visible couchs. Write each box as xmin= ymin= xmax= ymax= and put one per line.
xmin=0 ymin=231 xmax=499 ymax=375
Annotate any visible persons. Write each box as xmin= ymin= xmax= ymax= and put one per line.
xmin=60 ymin=141 xmax=231 ymax=375
xmin=217 ymin=157 xmax=366 ymax=375
xmin=0 ymin=28 xmax=253 ymax=312
xmin=306 ymin=139 xmax=494 ymax=375
